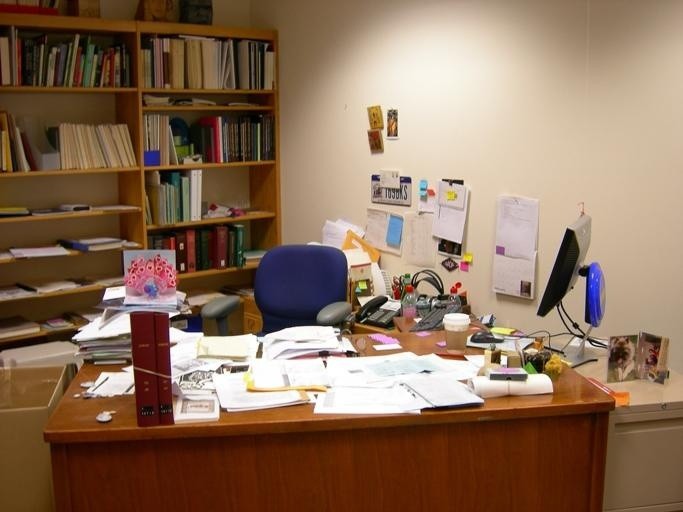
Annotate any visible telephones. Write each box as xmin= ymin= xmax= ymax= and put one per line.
xmin=356 ymin=295 xmax=402 ymax=327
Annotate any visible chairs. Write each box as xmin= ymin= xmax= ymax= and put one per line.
xmin=197 ymin=244 xmax=352 ymax=340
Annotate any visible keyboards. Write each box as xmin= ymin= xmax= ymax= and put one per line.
xmin=408 ymin=299 xmax=458 ymax=331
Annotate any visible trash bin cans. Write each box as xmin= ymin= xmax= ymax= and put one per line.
xmin=0 ymin=362 xmax=78 ymax=512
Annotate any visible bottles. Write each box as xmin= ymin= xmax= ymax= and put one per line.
xmin=399 ymin=273 xmax=419 ymax=317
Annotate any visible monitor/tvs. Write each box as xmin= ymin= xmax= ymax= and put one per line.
xmin=536 ymin=214 xmax=593 ymax=318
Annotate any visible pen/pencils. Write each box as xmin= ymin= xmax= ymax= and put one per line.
xmin=93 ymin=377 xmax=109 ymax=392
xmin=126 ymin=383 xmax=135 ymax=392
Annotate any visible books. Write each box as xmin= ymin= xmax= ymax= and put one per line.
xmin=102 ymin=250 xmax=186 ymax=313
xmin=139 ymin=33 xmax=277 ymax=313
xmin=0 ymin=25 xmax=141 ymax=339
xmin=67 ymin=313 xmax=554 ymax=425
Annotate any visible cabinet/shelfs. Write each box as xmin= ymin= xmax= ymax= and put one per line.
xmin=1 ymin=15 xmax=282 ymax=355
xmin=575 ymin=352 xmax=683 ymax=512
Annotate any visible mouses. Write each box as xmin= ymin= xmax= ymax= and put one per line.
xmin=470 ymin=329 xmax=504 ymax=344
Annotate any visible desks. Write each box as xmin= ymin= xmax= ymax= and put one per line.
xmin=44 ymin=342 xmax=614 ymax=512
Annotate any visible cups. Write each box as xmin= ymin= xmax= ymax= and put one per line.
xmin=443 ymin=312 xmax=470 ymax=356
